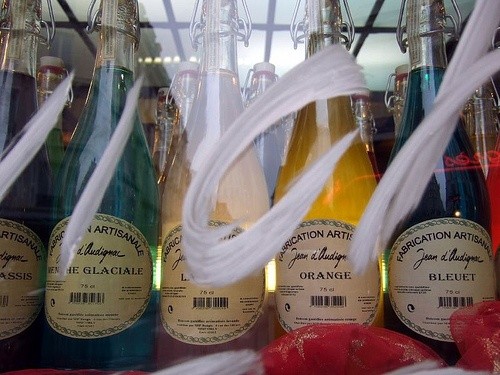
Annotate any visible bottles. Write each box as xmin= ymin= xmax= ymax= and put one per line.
xmin=1 ymin=0 xmax=500 ymax=373
